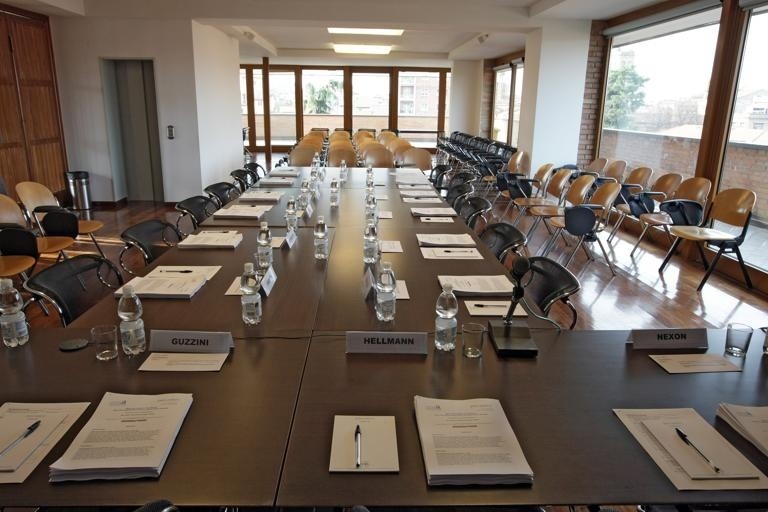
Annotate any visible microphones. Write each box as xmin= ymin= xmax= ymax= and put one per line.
xmin=511 ymin=255 xmax=530 ymax=300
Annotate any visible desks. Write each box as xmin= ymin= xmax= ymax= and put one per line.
xmin=67 ymin=226 xmax=336 ymax=330
xmin=274 ymin=331 xmax=766 ymax=512
xmin=0 ymin=331 xmax=311 ymax=510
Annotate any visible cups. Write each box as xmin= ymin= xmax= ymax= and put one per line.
xmin=460 ymin=323 xmax=486 ymax=359
xmin=762 ymin=328 xmax=768 ymax=354
xmin=90 ymin=324 xmax=118 ymax=360
xmin=713 ymin=352 xmax=746 ymax=394
xmin=725 ymin=323 xmax=755 ymax=357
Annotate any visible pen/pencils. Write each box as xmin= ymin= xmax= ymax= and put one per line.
xmin=675 ymin=427 xmax=720 ymax=473
xmin=203 ymin=231 xmax=229 ymax=233
xmin=354 ymin=424 xmax=361 ymax=468
xmin=0 ymin=420 xmax=41 ymax=457
xmin=444 ymin=250 xmax=472 ymax=252
xmin=425 ymin=218 xmax=448 ymax=220
xmin=160 ymin=270 xmax=192 ymax=273
xmin=474 ymin=304 xmax=507 ymax=307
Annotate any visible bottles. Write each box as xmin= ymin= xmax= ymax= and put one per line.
xmin=0 ymin=278 xmax=28 ymax=349
xmin=430 ymin=350 xmax=456 ymax=399
xmin=118 ymin=284 xmax=146 ymax=355
xmin=255 ymin=152 xmax=379 ymax=268
xmin=240 ymin=263 xmax=262 ymax=325
xmin=373 ymin=261 xmax=398 ymax=323
xmin=434 ymin=283 xmax=459 ymax=352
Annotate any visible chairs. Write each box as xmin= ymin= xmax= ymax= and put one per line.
xmin=174 ymin=195 xmax=220 ymax=236
xmin=429 ymin=132 xmax=627 ymax=276
xmin=242 ymin=127 xmax=254 ymax=159
xmin=630 ymin=177 xmax=713 ymax=273
xmin=608 ymin=167 xmax=653 ymax=242
xmin=658 ymin=188 xmax=757 ymax=294
xmin=23 ymin=253 xmax=125 ymax=328
xmin=245 ymin=163 xmax=266 ymax=184
xmin=14 ymin=181 xmax=106 ymax=292
xmin=610 ymin=173 xmax=682 ymax=256
xmin=204 ymin=182 xmax=241 ymax=206
xmin=288 ymin=127 xmax=432 ymax=171
xmin=230 ymin=168 xmax=257 ymax=193
xmin=0 ymin=194 xmax=88 ymax=317
xmin=120 ymin=218 xmax=182 ymax=276
xmin=510 ymin=257 xmax=582 ymax=331
xmin=1 ymin=256 xmax=48 ymax=330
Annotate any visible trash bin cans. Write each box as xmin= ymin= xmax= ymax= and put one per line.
xmin=66 ymin=171 xmax=92 ymax=210
